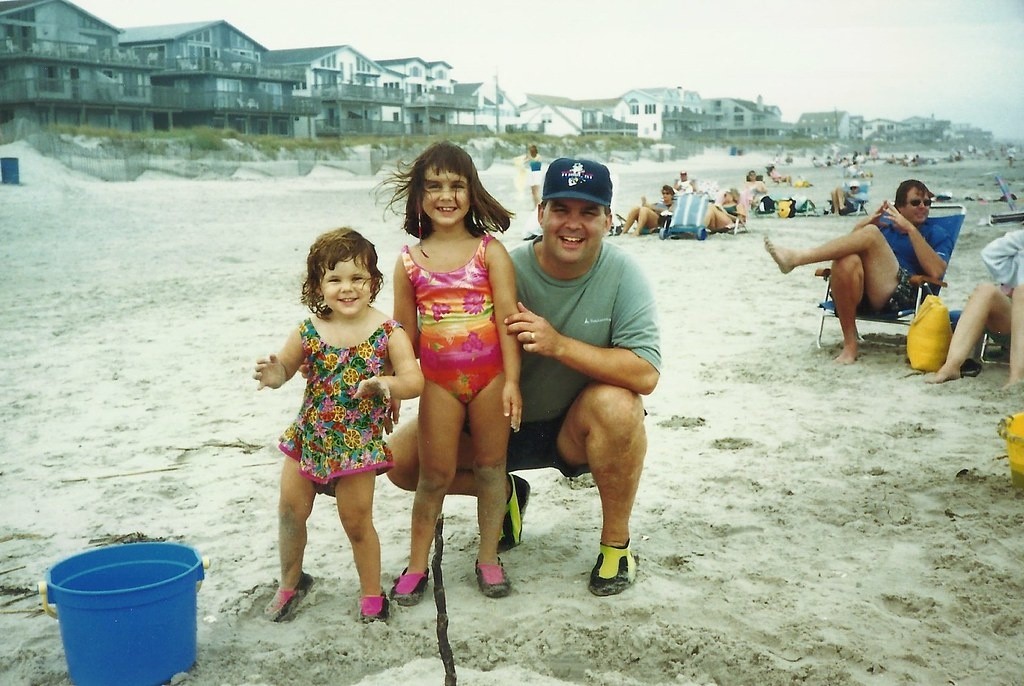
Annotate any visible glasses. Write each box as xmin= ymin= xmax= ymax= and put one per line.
xmin=908 ymin=200 xmax=931 ymax=206
xmin=663 ymin=192 xmax=670 ymax=195
xmin=724 ymin=194 xmax=726 ymax=197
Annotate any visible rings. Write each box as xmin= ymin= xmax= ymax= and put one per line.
xmin=531 ymin=332 xmax=536 ymax=343
xmin=893 ymin=214 xmax=896 ymax=217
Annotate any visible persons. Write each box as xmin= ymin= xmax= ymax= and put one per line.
xmin=519 ymin=146 xmax=541 ymax=212
xmin=299 ymin=157 xmax=664 ymax=596
xmin=764 ymin=180 xmax=953 ymax=365
xmin=924 ymin=229 xmax=1024 ymax=388
xmin=376 ymin=141 xmax=521 ymax=604
xmin=254 ymin=227 xmax=424 ymax=623
xmin=619 ymin=143 xmax=1017 ymax=237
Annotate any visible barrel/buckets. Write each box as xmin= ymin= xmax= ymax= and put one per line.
xmin=39 ymin=541 xmax=205 ymax=684
xmin=998 ymin=411 xmax=1024 ymax=492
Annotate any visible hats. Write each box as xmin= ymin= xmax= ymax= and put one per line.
xmin=850 ymin=181 xmax=861 ymax=188
xmin=681 ymin=172 xmax=687 ymax=175
xmin=542 ymin=158 xmax=612 ymax=206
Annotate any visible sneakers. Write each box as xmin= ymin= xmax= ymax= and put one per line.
xmin=359 ymin=587 xmax=389 ymax=623
xmin=476 ymin=556 xmax=510 ymax=598
xmin=590 ymin=539 xmax=636 ymax=596
xmin=391 ymin=566 xmax=429 ymax=606
xmin=265 ymin=572 xmax=314 ymax=623
xmin=497 ymin=474 xmax=531 ymax=554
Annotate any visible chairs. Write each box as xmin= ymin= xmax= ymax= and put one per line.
xmin=828 ymin=179 xmax=870 ymax=216
xmin=717 ymin=190 xmax=750 ymax=235
xmin=815 ymin=201 xmax=967 ymax=351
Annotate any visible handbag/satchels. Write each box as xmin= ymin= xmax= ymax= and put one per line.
xmin=907 ymin=295 xmax=953 ymax=373
